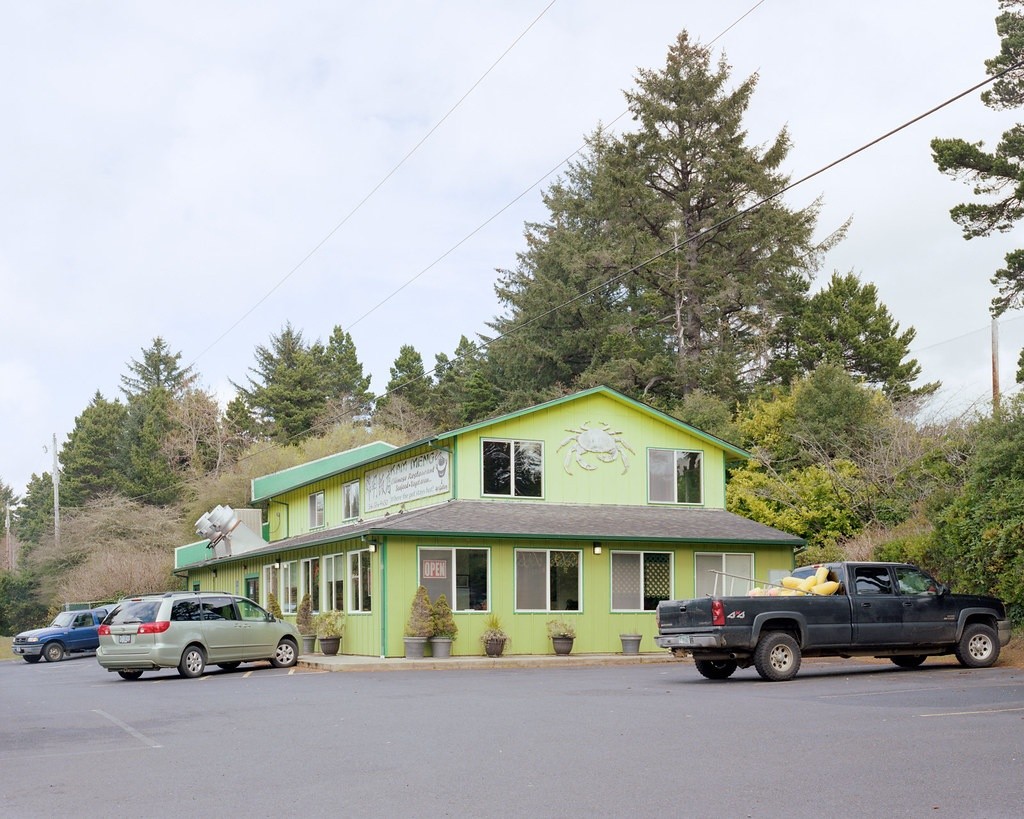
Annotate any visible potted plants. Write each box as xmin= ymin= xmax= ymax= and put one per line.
xmin=402 ymin=585 xmax=436 ymax=659
xmin=428 ymin=593 xmax=458 ymax=658
xmin=316 ymin=609 xmax=347 ymax=657
xmin=618 ymin=625 xmax=643 ymax=656
xmin=480 ymin=613 xmax=508 ymax=658
xmin=265 ymin=592 xmax=284 ymax=621
xmin=295 ymin=594 xmax=317 ymax=654
xmin=545 ymin=619 xmax=577 ymax=656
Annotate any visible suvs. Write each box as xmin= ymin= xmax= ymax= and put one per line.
xmin=96 ymin=591 xmax=307 ymax=677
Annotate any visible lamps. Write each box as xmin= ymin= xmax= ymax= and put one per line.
xmin=592 ymin=541 xmax=602 ymax=555
xmin=368 ymin=537 xmax=378 ymax=552
xmin=212 ymin=569 xmax=217 ymax=578
xmin=275 ymin=558 xmax=280 ymax=569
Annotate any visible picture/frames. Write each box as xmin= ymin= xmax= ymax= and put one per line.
xmin=457 ymin=573 xmax=470 ymax=588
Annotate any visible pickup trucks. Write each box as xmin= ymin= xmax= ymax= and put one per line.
xmin=653 ymin=561 xmax=1013 ymax=682
xmin=11 ymin=608 xmax=111 ymax=664
